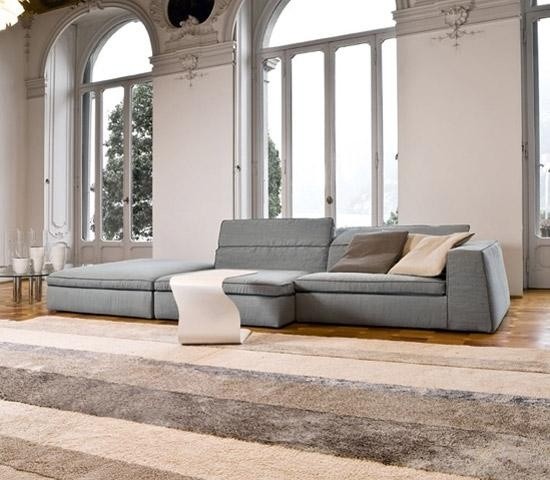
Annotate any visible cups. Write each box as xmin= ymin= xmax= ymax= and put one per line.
xmin=50 ymin=243 xmax=66 ymax=271
xmin=29 ymin=246 xmax=45 ymax=273
xmin=12 ymin=257 xmax=29 ymax=275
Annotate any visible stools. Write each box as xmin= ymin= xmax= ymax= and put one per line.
xmin=46 ymin=256 xmax=214 ymax=319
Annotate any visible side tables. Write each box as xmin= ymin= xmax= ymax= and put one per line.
xmin=0 ymin=265 xmax=57 ymax=305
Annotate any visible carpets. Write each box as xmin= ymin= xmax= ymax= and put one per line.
xmin=0 ymin=314 xmax=550 ymax=480
